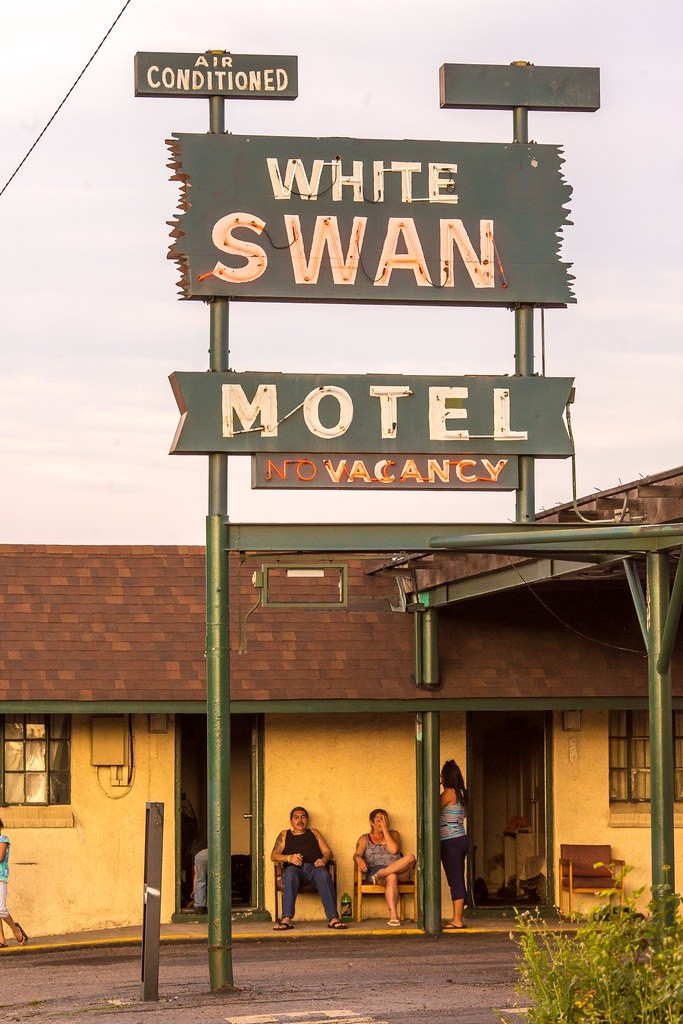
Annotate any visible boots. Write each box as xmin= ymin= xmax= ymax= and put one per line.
xmin=372 ymin=874 xmax=378 ymax=886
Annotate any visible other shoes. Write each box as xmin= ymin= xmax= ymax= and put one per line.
xmin=194 ymin=906 xmax=207 ymax=914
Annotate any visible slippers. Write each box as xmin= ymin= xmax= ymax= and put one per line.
xmin=0 ymin=943 xmax=8 ymax=947
xmin=386 ymin=920 xmax=401 ymax=927
xmin=273 ymin=921 xmax=294 ymax=930
xmin=442 ymin=921 xmax=467 ymax=929
xmin=328 ymin=922 xmax=347 ymax=929
xmin=14 ymin=923 xmax=29 ymax=945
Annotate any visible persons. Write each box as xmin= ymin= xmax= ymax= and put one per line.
xmin=183 ymin=833 xmax=208 ymax=914
xmin=271 ymin=807 xmax=348 ymax=931
xmin=0 ymin=818 xmax=29 ymax=948
xmin=440 ymin=759 xmax=470 ymax=929
xmin=353 ymin=809 xmax=415 ymax=926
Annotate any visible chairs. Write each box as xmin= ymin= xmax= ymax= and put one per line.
xmin=354 ymin=861 xmax=418 ymax=923
xmin=274 ymin=861 xmax=337 ymax=923
xmin=559 ymin=844 xmax=626 ymax=924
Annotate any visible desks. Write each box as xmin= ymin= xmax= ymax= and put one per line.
xmin=502 ymin=831 xmax=534 ymax=901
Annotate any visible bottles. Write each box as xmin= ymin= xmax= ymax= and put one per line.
xmin=340 ymin=892 xmax=353 ymax=921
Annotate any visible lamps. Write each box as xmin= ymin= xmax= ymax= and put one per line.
xmin=149 ymin=713 xmax=167 ymax=733
xmin=563 ymin=710 xmax=581 ymax=732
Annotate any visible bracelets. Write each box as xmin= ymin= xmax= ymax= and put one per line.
xmin=288 ymin=855 xmax=292 ymax=862
